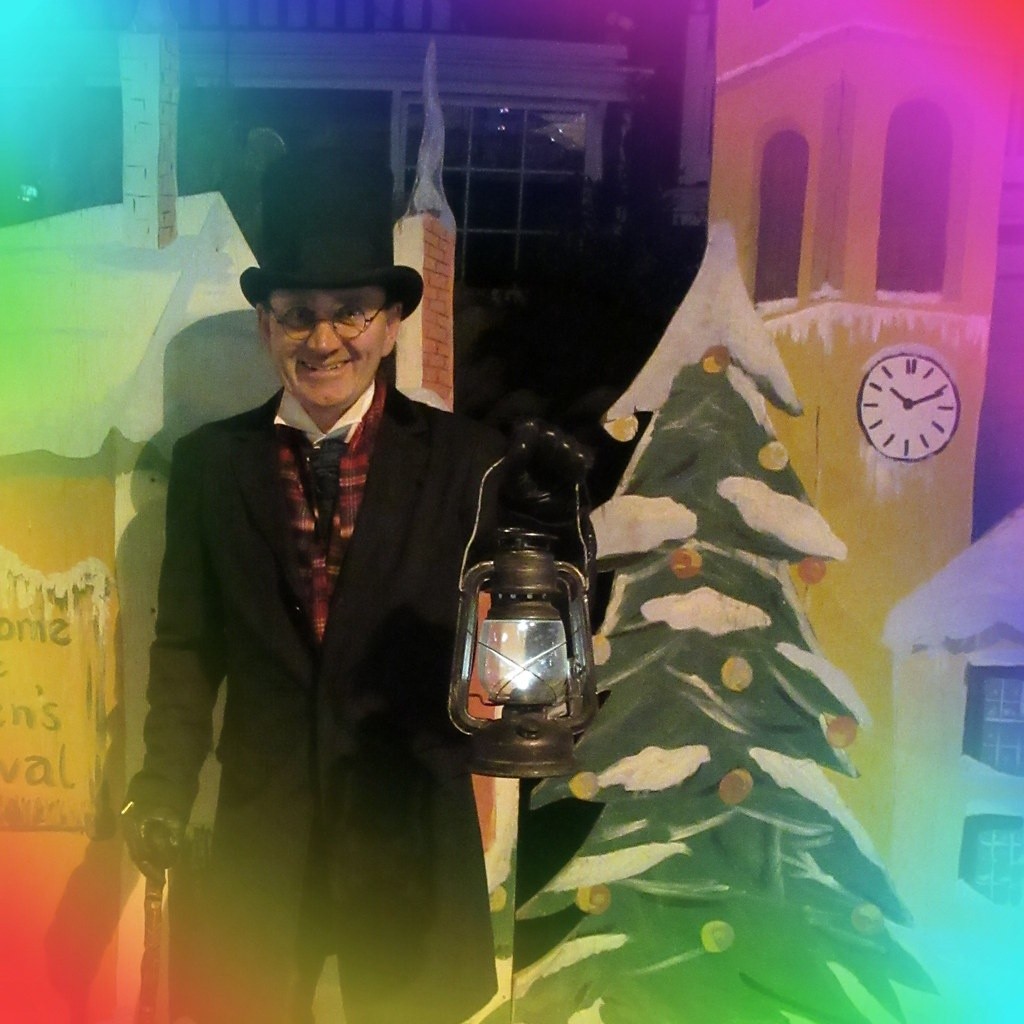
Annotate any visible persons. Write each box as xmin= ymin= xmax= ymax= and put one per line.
xmin=121 ymin=151 xmax=595 ymax=1024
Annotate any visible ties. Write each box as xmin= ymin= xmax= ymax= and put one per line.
xmin=289 ymin=434 xmax=347 ymax=550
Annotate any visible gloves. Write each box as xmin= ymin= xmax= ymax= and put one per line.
xmin=506 ymin=423 xmax=590 ymax=528
xmin=113 ymin=772 xmax=192 ymax=882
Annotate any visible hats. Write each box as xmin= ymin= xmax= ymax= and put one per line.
xmin=239 ymin=132 xmax=425 ymax=321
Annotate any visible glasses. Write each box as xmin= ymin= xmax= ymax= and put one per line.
xmin=265 ymin=300 xmax=389 ymax=340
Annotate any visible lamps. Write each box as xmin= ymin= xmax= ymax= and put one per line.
xmin=447 ymin=450 xmax=600 ymax=778
